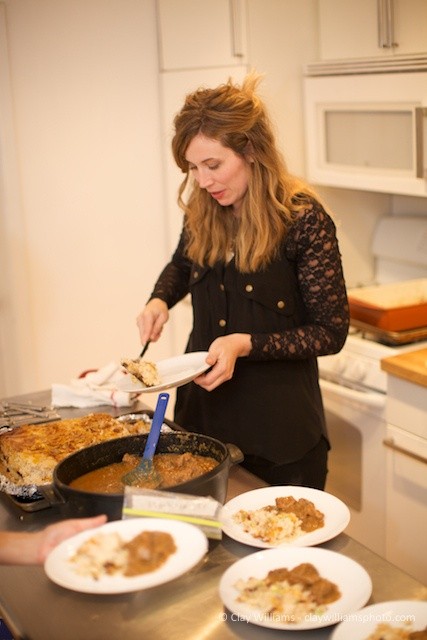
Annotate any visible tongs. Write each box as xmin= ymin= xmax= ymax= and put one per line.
xmin=1 ymin=402 xmax=54 ymax=419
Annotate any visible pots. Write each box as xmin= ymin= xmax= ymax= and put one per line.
xmin=39 ymin=432 xmax=244 ymax=522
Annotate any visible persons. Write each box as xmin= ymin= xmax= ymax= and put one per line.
xmin=136 ymin=79 xmax=349 ymax=492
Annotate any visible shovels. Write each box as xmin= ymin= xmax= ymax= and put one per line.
xmin=122 ymin=339 xmax=156 ymax=388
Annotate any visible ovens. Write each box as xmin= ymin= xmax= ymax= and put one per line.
xmin=317 ymin=349 xmax=388 ymax=559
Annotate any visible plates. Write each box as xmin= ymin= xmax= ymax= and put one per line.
xmin=218 ymin=547 xmax=372 ymax=631
xmin=217 ymin=486 xmax=350 ymax=548
xmin=330 ymin=600 xmax=426 ymax=640
xmin=44 ymin=518 xmax=208 ymax=595
xmin=112 ymin=351 xmax=211 ymax=393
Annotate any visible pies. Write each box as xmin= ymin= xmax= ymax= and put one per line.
xmin=1 ymin=414 xmax=152 ymax=490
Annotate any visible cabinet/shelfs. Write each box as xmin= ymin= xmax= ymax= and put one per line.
xmin=300 ymin=69 xmax=427 ymax=196
xmin=304 ymin=0 xmax=427 ymax=62
xmin=380 ymin=374 xmax=426 ymax=594
xmin=156 ymin=0 xmax=302 ymax=67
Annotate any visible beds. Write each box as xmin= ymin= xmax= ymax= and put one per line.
xmin=0 ymin=387 xmax=427 ymax=640
